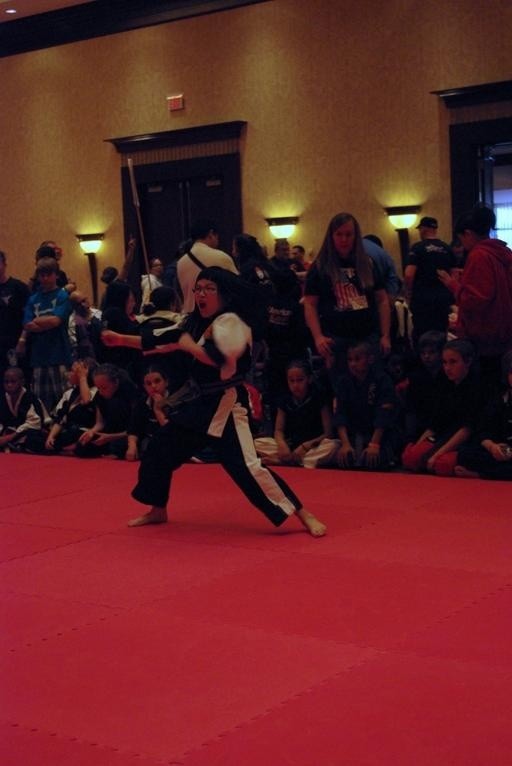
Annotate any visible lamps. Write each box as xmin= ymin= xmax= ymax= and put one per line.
xmin=77 ymin=232 xmax=107 ymax=256
xmin=385 ymin=203 xmax=423 ymax=234
xmin=264 ymin=213 xmax=300 ymax=238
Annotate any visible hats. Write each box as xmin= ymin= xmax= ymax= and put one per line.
xmin=415 ymin=216 xmax=438 ymax=230
